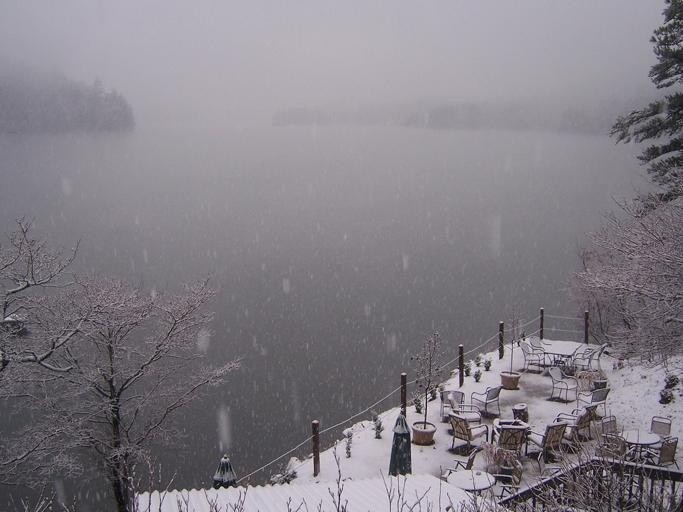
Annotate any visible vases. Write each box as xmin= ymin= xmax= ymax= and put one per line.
xmin=411 ymin=421 xmax=437 ymax=446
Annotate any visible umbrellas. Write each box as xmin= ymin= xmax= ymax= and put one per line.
xmin=387 ymin=406 xmax=414 ymax=476
xmin=209 ymin=449 xmax=239 ymax=491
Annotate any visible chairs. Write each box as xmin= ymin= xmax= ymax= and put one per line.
xmin=440 ymin=335 xmax=679 ymax=498
